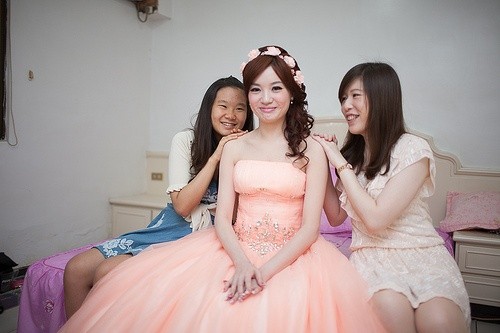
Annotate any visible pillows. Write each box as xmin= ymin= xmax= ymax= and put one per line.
xmin=441 ymin=190 xmax=500 ymax=232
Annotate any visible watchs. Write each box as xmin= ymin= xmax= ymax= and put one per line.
xmin=338 ymin=162 xmax=353 ymax=174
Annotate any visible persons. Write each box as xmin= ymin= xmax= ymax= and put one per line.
xmin=312 ymin=62 xmax=471 ymax=333
xmin=55 ymin=45 xmax=386 ymax=333
xmin=63 ymin=76 xmax=254 ymax=321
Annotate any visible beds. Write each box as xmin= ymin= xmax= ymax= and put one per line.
xmin=18 ymin=117 xmax=500 ymax=333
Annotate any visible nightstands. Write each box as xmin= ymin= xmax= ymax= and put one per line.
xmin=108 ymin=192 xmax=172 ymax=236
xmin=453 ymin=230 xmax=500 ymax=321
